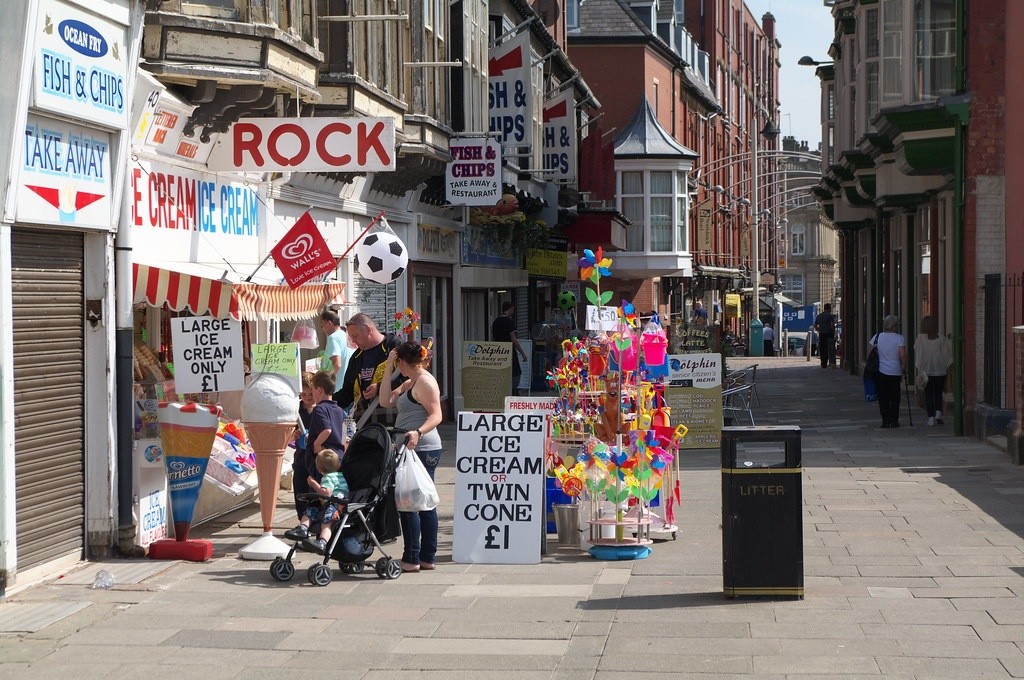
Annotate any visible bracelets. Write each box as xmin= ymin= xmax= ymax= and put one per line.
xmin=416 ymin=429 xmax=423 ymax=440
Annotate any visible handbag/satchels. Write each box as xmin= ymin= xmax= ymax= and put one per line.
xmin=395 ymin=443 xmax=440 ymax=513
xmin=864 ymin=332 xmax=879 ymax=377
xmin=916 ymin=370 xmax=929 ymax=391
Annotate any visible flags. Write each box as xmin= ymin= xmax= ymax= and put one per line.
xmin=271 ymin=213 xmax=337 ymax=290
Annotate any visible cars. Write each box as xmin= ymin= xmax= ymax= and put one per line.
xmin=781 ymin=327 xmax=842 ymax=357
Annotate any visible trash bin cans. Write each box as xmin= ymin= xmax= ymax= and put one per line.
xmin=720 ymin=424 xmax=806 ymax=601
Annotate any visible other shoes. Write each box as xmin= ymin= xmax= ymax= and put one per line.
xmin=935 ymin=410 xmax=944 ymax=424
xmin=302 ymin=539 xmax=327 ymax=556
xmin=881 ymin=422 xmax=890 ymax=428
xmin=284 ymin=525 xmax=309 ymax=540
xmin=380 ymin=538 xmax=398 ymax=546
xmin=926 ymin=417 xmax=935 ymax=426
xmin=891 ymin=421 xmax=899 ymax=428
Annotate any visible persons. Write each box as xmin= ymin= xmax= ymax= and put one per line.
xmin=764 ymin=322 xmax=775 ymax=356
xmin=554 ymin=309 xmax=571 ymax=331
xmin=869 ymin=316 xmax=908 ymax=429
xmin=307 ymin=375 xmax=342 ymax=492
xmin=291 ymin=370 xmax=317 ymax=537
xmin=284 ymin=448 xmax=349 ymax=556
xmin=381 ymin=345 xmax=443 ymax=573
xmin=492 ymin=301 xmax=527 ymax=396
xmin=914 ymin=316 xmax=954 ymax=426
xmin=342 ymin=311 xmax=406 ymax=423
xmin=813 ymin=303 xmax=835 ymax=368
xmin=320 ymin=310 xmax=357 ymax=409
xmin=640 ymin=310 xmax=656 ymax=328
xmin=805 ymin=325 xmax=818 ymax=356
xmin=695 ymin=301 xmax=708 ymax=324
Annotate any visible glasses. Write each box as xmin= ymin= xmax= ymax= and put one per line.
xmin=396 ymin=355 xmax=403 ymax=364
xmin=345 ymin=320 xmax=367 ymax=328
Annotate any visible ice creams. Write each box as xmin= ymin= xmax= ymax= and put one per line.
xmin=240 ymin=371 xmax=300 ymax=532
xmin=157 ymin=400 xmax=224 ymax=541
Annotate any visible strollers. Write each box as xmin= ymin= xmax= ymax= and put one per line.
xmin=269 ymin=421 xmax=410 ymax=588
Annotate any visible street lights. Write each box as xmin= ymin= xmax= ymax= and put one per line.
xmin=748 ymin=108 xmax=782 ymax=356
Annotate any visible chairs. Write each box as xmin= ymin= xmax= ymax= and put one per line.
xmin=720 ymin=364 xmax=760 ymax=428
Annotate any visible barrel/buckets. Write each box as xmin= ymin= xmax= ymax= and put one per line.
xmin=643 ymin=324 xmax=669 ymax=365
xmin=588 ymin=346 xmax=607 ymax=375
xmin=610 ymin=337 xmax=640 ymax=371
xmin=636 ymin=396 xmax=675 ymax=449
xmin=551 ymin=502 xmax=584 ymax=545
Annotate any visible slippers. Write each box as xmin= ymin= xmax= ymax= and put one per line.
xmin=419 ymin=557 xmax=436 ymax=570
xmin=398 ymin=559 xmax=420 ymax=573
